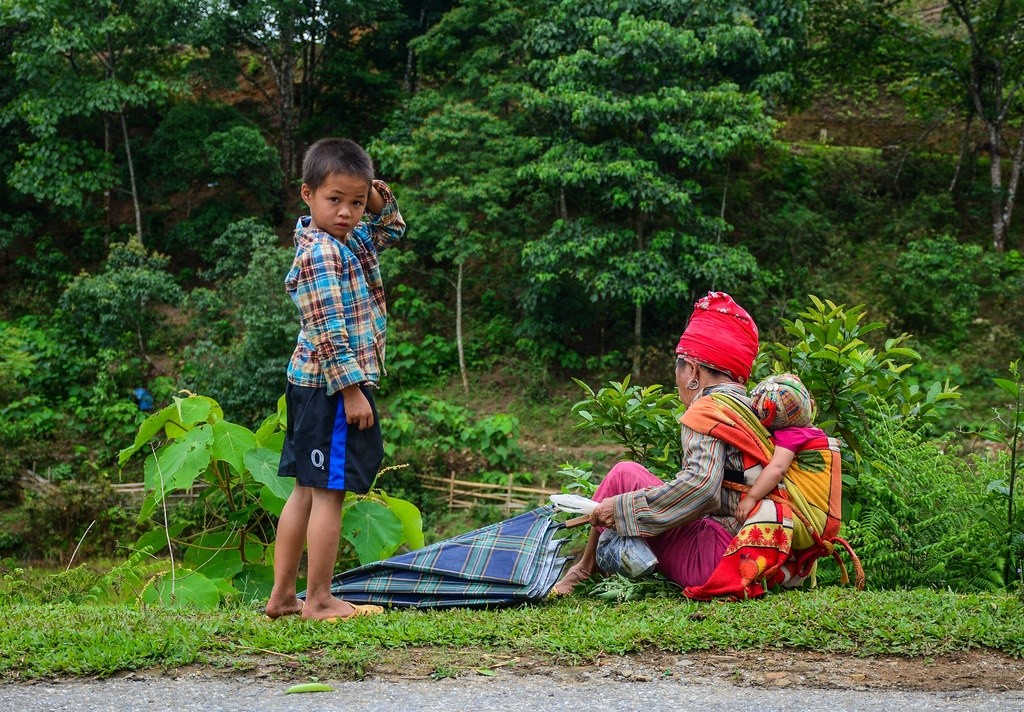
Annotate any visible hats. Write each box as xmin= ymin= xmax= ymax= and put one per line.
xmin=676 ymin=291 xmax=760 ymax=384
xmin=748 ymin=372 xmax=813 ymax=427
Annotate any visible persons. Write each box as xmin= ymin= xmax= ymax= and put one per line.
xmin=733 ymin=375 xmax=842 ymax=567
xmin=541 ymin=291 xmax=791 ymax=599
xmin=262 ymin=139 xmax=407 ymax=622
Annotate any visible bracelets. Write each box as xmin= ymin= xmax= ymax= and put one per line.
xmin=589 ymin=514 xmax=597 ymax=519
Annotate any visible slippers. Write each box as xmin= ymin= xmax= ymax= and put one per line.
xmin=266 ymin=599 xmax=305 ymax=622
xmin=322 ymin=598 xmax=384 ymax=623
